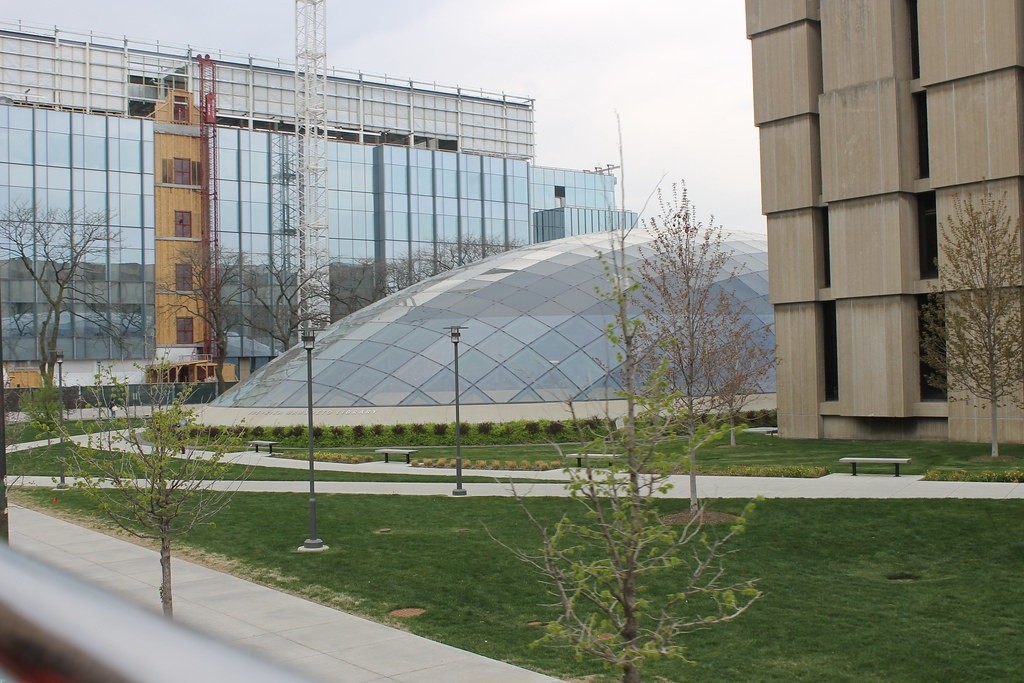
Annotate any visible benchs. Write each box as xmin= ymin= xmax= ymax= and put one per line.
xmin=565 ymin=453 xmax=625 ymax=468
xmin=374 ymin=448 xmax=420 ymax=464
xmin=247 ymin=440 xmax=280 ymax=454
xmin=839 ymin=457 xmax=913 ymax=478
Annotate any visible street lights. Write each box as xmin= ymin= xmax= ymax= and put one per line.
xmin=292 ymin=326 xmax=330 ymax=548
xmin=444 ymin=325 xmax=471 ymax=494
xmin=51 ymin=350 xmax=68 ymax=488
